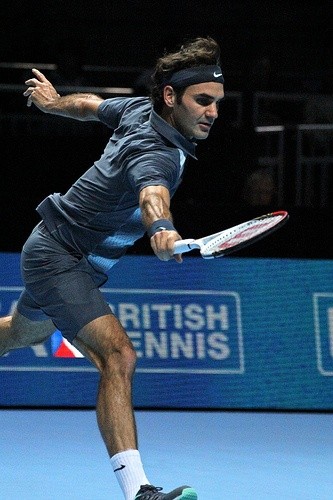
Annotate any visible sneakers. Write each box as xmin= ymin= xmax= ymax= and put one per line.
xmin=135 ymin=485 xmax=198 ymax=500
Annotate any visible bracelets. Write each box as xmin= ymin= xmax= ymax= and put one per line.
xmin=146 ymin=218 xmax=176 ymax=239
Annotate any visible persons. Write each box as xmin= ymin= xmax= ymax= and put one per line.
xmin=216 ymin=167 xmax=278 ymax=258
xmin=1 ymin=38 xmax=223 ymax=500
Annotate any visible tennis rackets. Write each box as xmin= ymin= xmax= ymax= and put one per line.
xmin=172 ymin=209 xmax=290 ymax=259
